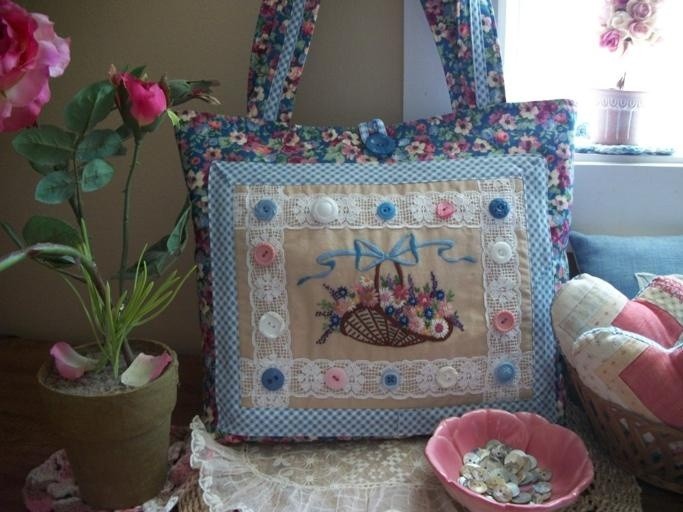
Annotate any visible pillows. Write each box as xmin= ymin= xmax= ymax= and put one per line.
xmin=165 ymin=95 xmax=682 ymax=453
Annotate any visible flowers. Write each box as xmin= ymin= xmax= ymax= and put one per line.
xmin=586 ymin=0 xmax=660 ymax=92
xmin=0 ymin=2 xmax=223 ymax=375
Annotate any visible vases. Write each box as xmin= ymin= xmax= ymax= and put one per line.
xmin=36 ymin=338 xmax=179 ymax=511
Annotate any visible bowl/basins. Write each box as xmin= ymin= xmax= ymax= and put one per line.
xmin=424 ymin=408 xmax=595 ymax=512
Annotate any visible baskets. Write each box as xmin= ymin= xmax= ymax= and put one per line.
xmin=567 ymin=365 xmax=683 ymax=494
